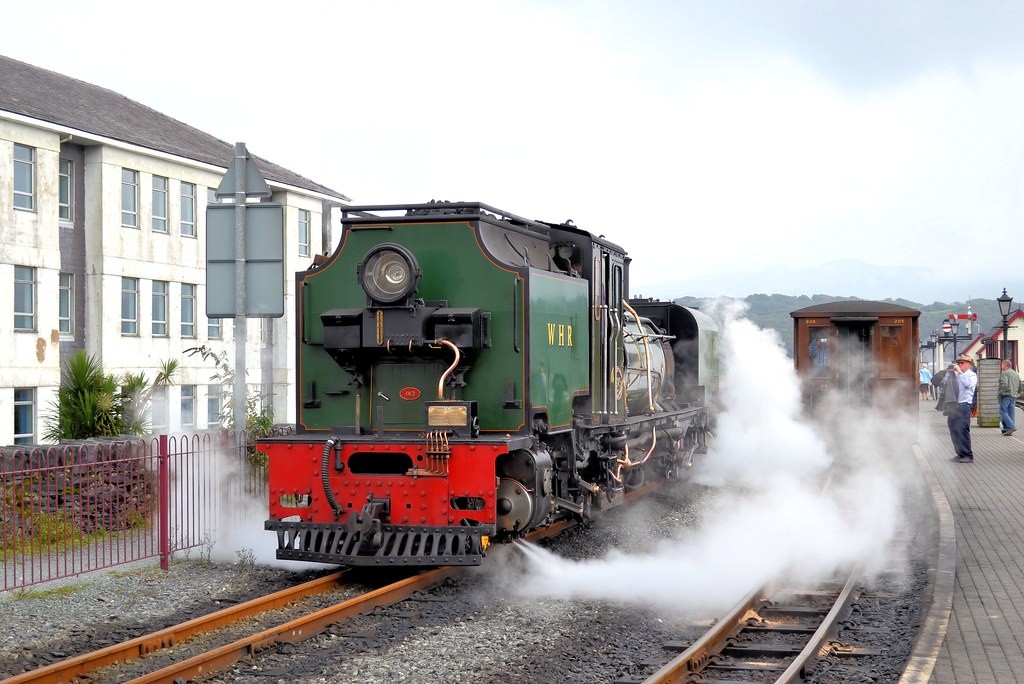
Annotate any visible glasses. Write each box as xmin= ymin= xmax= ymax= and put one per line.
xmin=958 ymin=361 xmax=970 ymax=364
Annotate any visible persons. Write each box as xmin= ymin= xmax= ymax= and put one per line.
xmin=997 ymin=360 xmax=1024 ymax=437
xmin=808 ymin=322 xmax=838 ymax=381
xmin=918 ymin=363 xmax=933 ymax=402
xmin=947 ymin=355 xmax=979 ymax=462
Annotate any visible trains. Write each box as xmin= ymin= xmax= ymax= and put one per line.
xmin=788 ymin=299 xmax=921 ymax=427
xmin=254 ymin=196 xmax=728 ymax=586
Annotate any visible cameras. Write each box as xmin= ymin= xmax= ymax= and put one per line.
xmin=949 ymin=364 xmax=955 ymax=369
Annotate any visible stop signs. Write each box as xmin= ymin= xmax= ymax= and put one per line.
xmin=942 ymin=323 xmax=952 ymax=334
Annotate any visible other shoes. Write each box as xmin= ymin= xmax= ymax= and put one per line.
xmin=948 ymin=454 xmax=963 ymax=462
xmin=1002 ymin=428 xmax=1017 ymax=436
xmin=959 ymin=455 xmax=973 ymax=463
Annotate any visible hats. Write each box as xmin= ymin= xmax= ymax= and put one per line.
xmin=956 ymin=354 xmax=974 ymax=365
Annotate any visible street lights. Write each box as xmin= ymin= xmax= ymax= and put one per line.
xmin=996 ymin=286 xmax=1014 ymax=361
xmin=950 ymin=318 xmax=960 ymax=361
xmin=930 ymin=330 xmax=937 ymax=377
